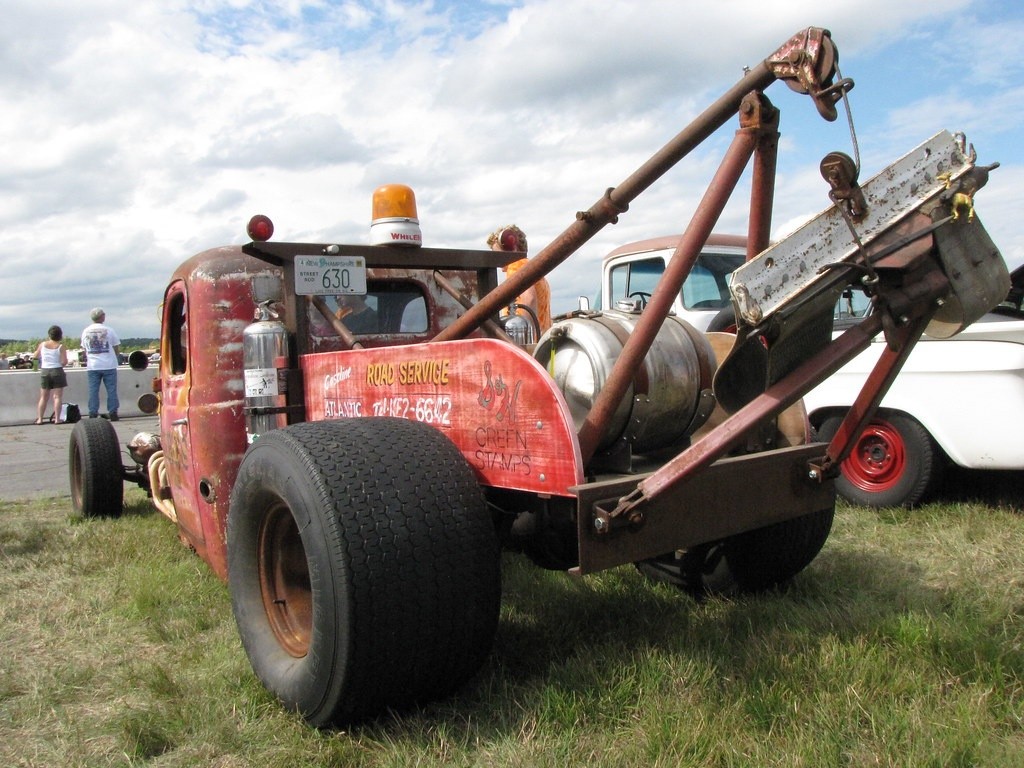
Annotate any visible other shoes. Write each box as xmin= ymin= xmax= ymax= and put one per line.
xmin=111 ymin=417 xmax=119 ymax=421
xmin=89 ymin=415 xmax=98 ymax=418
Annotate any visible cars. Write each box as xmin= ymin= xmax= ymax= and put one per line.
xmin=599 ymin=238 xmax=1024 ymax=516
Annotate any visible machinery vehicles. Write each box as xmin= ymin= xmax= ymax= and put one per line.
xmin=66 ymin=28 xmax=1013 ymax=729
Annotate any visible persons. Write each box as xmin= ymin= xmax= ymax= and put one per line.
xmin=324 ymin=294 xmax=377 ymax=335
xmin=0 ymin=351 xmax=31 ymax=370
xmin=29 ymin=325 xmax=70 ymax=426
xmin=80 ymin=308 xmax=121 ymax=422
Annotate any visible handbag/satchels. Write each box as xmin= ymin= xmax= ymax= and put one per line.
xmin=60 ymin=403 xmax=81 ymax=423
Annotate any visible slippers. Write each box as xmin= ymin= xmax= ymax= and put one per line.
xmin=54 ymin=420 xmax=66 ymax=424
xmin=33 ymin=419 xmax=43 ymax=425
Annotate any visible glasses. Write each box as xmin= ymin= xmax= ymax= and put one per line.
xmin=102 ymin=313 xmax=105 ymax=315
